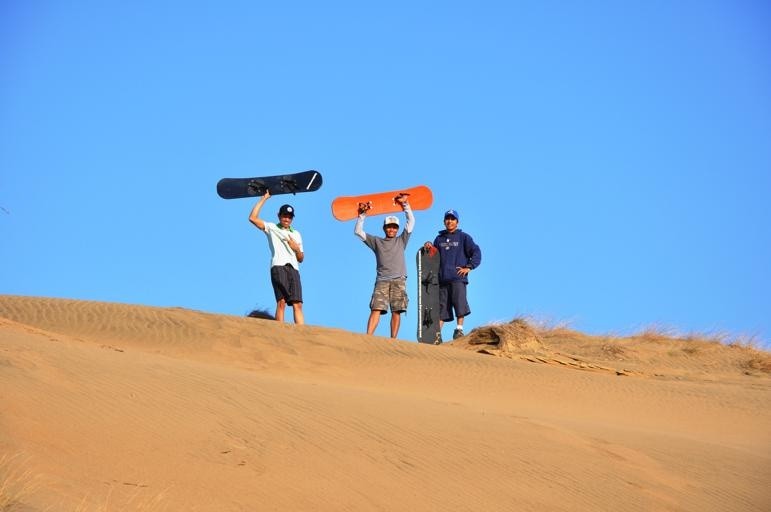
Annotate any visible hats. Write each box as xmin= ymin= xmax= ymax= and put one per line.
xmin=384 ymin=216 xmax=400 ymax=228
xmin=445 ymin=210 xmax=459 ymax=222
xmin=280 ymin=205 xmax=295 ymax=217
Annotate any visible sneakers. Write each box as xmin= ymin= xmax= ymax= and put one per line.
xmin=453 ymin=330 xmax=464 ymax=339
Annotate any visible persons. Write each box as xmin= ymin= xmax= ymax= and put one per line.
xmin=355 ymin=193 xmax=416 ymax=338
xmin=425 ymin=208 xmax=482 ymax=339
xmin=249 ymin=189 xmax=305 ymax=326
